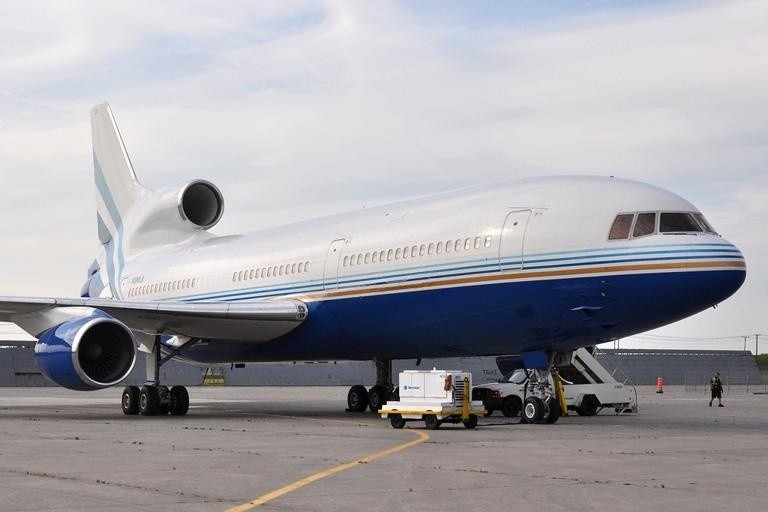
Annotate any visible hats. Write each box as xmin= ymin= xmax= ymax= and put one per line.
xmin=716 ymin=371 xmax=721 ymax=375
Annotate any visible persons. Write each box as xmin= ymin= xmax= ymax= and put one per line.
xmin=709 ymin=371 xmax=724 ymax=407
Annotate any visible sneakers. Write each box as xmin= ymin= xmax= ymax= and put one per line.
xmin=708 ymin=401 xmax=725 ymax=408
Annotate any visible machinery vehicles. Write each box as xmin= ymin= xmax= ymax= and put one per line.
xmin=471 ymin=345 xmax=638 ymax=420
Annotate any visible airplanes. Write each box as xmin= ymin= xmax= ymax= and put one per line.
xmin=0 ymin=96 xmax=754 ymax=419
